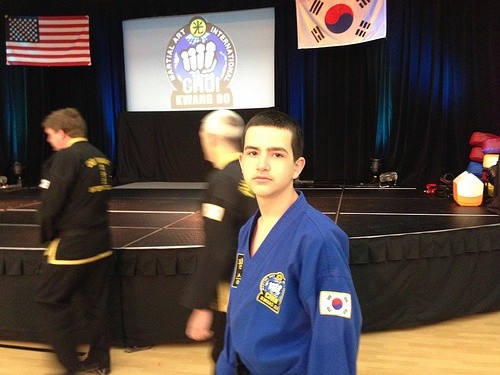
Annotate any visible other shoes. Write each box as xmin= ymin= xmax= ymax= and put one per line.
xmin=81 ymin=358 xmax=110 ymax=375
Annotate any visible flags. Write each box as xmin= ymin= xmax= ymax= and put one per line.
xmin=296 ymin=0 xmax=387 ymax=49
xmin=4 ymin=15 xmax=91 ymax=66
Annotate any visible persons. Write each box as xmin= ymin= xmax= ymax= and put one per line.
xmin=217 ymin=111 xmax=363 ymax=375
xmin=185 ymin=110 xmax=259 ymax=375
xmin=24 ymin=108 xmax=113 ymax=375
